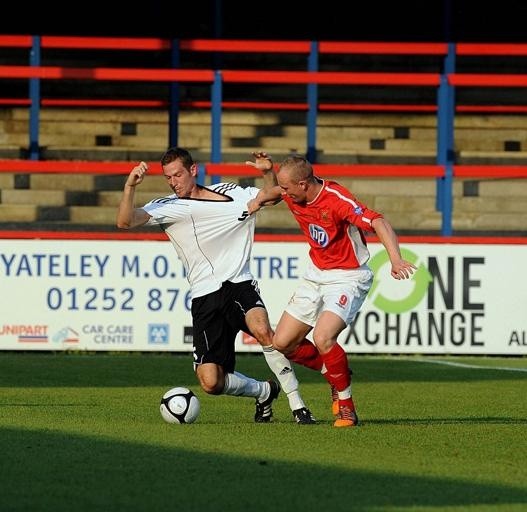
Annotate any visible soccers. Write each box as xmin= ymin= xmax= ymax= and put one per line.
xmin=160 ymin=386 xmax=201 ymax=424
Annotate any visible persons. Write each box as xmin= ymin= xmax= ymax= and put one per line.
xmin=115 ymin=146 xmax=318 ymax=425
xmin=245 ymin=155 xmax=417 ymax=427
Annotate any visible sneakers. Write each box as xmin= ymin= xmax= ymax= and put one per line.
xmin=293 ymin=407 xmax=317 ymax=425
xmin=254 ymin=378 xmax=281 ymax=423
xmin=332 ymin=368 xmax=353 ymax=417
xmin=334 ymin=405 xmax=358 ymax=428
